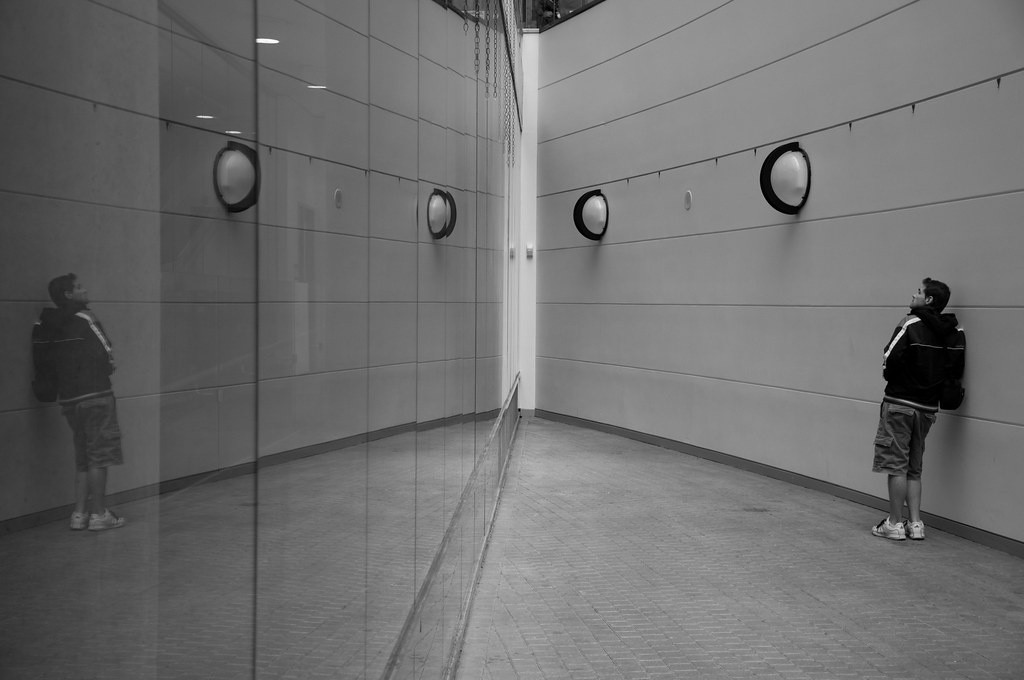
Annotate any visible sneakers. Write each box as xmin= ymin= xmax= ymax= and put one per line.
xmin=871 ymin=515 xmax=906 ymax=539
xmin=902 ymin=518 xmax=925 ymax=539
xmin=88 ymin=509 xmax=125 ymax=530
xmin=70 ymin=511 xmax=89 ymax=529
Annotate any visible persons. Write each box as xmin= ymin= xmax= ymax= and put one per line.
xmin=872 ymin=277 xmax=967 ymax=541
xmin=31 ymin=272 xmax=126 ymax=530
